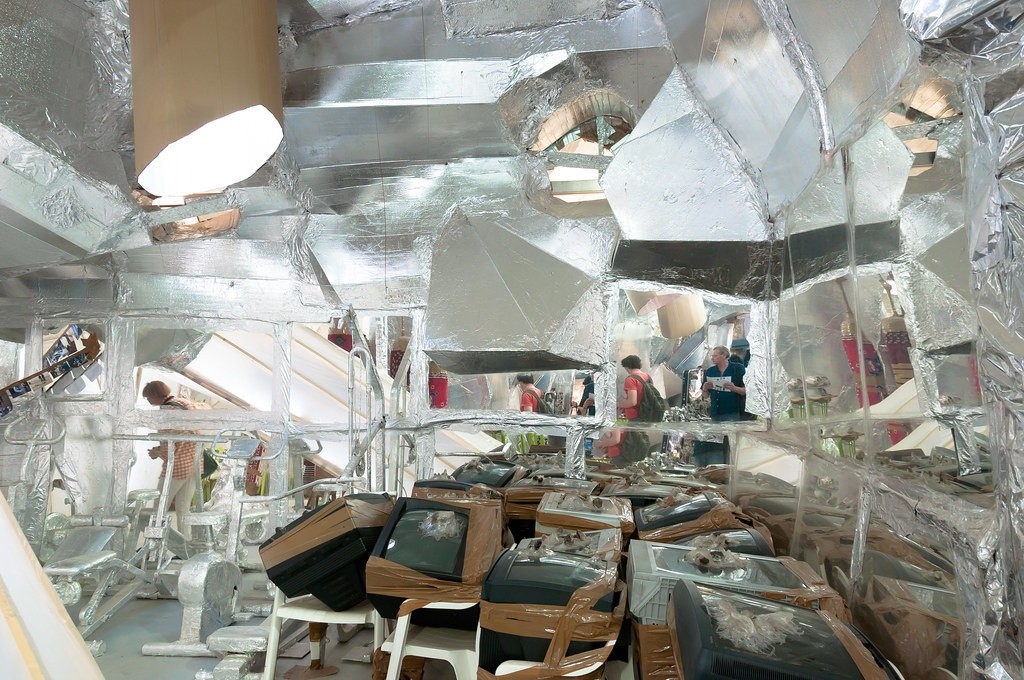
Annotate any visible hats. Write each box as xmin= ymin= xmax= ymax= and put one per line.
xmin=731 ymin=338 xmax=750 ymax=350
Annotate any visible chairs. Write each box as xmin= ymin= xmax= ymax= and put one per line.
xmin=495 ymin=579 xmax=628 ymax=680
xmin=264 ymin=539 xmax=395 ymax=680
xmin=606 ymin=621 xmax=641 ymax=680
xmin=381 ymin=543 xmax=517 ymax=680
xmin=831 ymin=565 xmax=851 ymax=603
xmin=742 ymin=507 xmax=790 ymax=556
xmin=804 ymin=540 xmax=821 ymax=576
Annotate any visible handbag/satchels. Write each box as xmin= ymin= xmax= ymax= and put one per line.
xmin=200 ymin=449 xmax=219 ymax=480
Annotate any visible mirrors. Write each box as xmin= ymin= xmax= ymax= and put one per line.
xmin=43 ymin=270 xmax=958 ymax=680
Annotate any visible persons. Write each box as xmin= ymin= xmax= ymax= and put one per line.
xmin=517 ymin=372 xmax=542 ymax=413
xmin=700 ymin=345 xmax=758 ymax=420
xmin=590 ymin=426 xmax=727 ymax=469
xmin=617 ymin=355 xmax=654 ymax=427
xmin=136 ymin=382 xmax=204 ymax=561
xmin=571 ymin=372 xmax=594 ymax=416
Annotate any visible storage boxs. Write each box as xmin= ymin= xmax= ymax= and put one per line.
xmin=534 ymin=492 xmax=634 ymax=547
xmin=631 ymin=619 xmax=681 ymax=680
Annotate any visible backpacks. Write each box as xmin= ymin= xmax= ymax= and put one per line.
xmin=624 ymin=372 xmax=667 ymax=423
xmin=610 ymin=420 xmax=651 ymax=464
xmin=735 ymin=362 xmax=758 ymax=421
xmin=521 ymin=389 xmax=553 ymax=415
